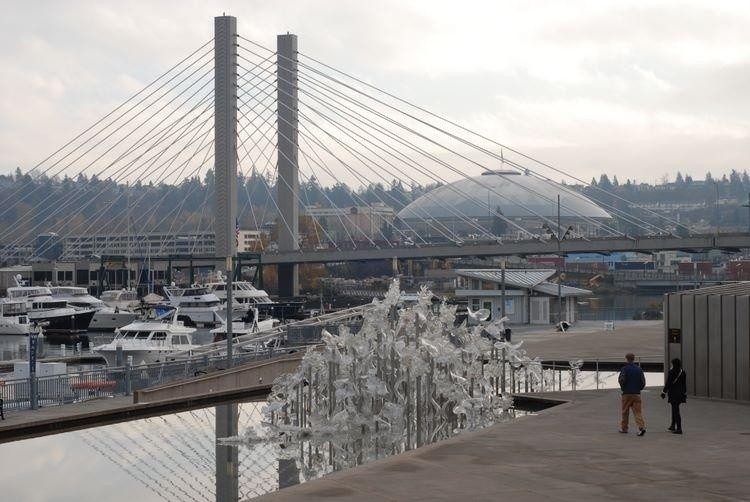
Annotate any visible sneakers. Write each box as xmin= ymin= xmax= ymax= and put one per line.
xmin=638 ymin=429 xmax=647 ymax=436
xmin=619 ymin=429 xmax=628 ymax=434
xmin=668 ymin=426 xmax=676 ymax=431
xmin=673 ymin=429 xmax=684 ymax=435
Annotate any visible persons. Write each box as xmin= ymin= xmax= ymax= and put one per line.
xmin=617 ymin=352 xmax=647 ymax=436
xmin=660 ymin=358 xmax=689 ymax=434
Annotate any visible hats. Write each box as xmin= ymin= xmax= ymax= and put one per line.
xmin=626 ymin=352 xmax=635 ymax=360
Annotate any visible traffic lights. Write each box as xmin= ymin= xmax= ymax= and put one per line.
xmin=543 ymin=223 xmax=558 ymax=243
xmin=562 ymin=225 xmax=573 ymax=241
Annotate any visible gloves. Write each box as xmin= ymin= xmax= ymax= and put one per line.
xmin=683 ymin=394 xmax=687 ymax=403
xmin=660 ymin=392 xmax=667 ymax=399
